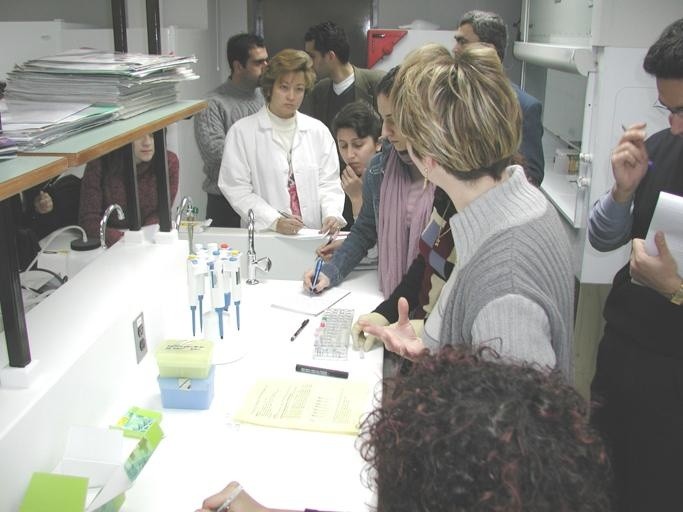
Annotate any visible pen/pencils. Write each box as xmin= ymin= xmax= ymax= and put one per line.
xmin=621 ymin=124 xmax=657 ymax=172
xmin=291 ymin=319 xmax=309 ymax=341
xmin=310 ymin=258 xmax=323 ymax=298
xmin=278 ymin=210 xmax=306 ymax=226
xmin=215 ymin=484 xmax=245 ymax=512
xmin=314 ymin=238 xmax=334 ymax=263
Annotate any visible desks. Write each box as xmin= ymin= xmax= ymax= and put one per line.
xmin=1 ymin=223 xmax=390 ymax=512
xmin=15 ymin=100 xmax=210 ymax=233
xmin=0 ymin=155 xmax=68 ymax=368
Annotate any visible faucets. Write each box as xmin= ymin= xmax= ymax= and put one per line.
xmin=245 ymin=207 xmax=273 ymax=288
xmin=170 ymin=196 xmax=214 ymax=258
xmin=98 ymin=197 xmax=125 ymax=255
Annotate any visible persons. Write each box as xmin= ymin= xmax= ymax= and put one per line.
xmin=302 ymin=65 xmax=435 ymax=299
xmin=298 ymin=21 xmax=389 ymax=230
xmin=13 ymin=174 xmax=81 ymax=272
xmin=77 ymin=127 xmax=179 ymax=247
xmin=195 ymin=336 xmax=615 ymax=511
xmin=218 ymin=48 xmax=348 ymax=235
xmin=587 ymin=17 xmax=683 ymax=512
xmin=315 ymin=100 xmax=383 ymax=262
xmin=451 ymin=10 xmax=545 ymax=186
xmin=194 ymin=33 xmax=269 ymax=229
xmin=362 ymin=44 xmax=575 ymax=395
xmin=351 ymin=155 xmax=539 ymax=351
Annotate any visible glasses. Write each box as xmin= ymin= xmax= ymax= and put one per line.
xmin=653 ymin=99 xmax=683 ymax=122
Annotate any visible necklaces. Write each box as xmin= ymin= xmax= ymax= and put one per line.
xmin=434 ymin=201 xmax=452 ymax=248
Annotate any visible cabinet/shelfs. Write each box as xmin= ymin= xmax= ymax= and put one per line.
xmin=513 ymin=39 xmax=595 ymax=284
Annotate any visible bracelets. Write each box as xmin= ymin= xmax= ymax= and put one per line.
xmin=670 ymin=275 xmax=683 ymax=305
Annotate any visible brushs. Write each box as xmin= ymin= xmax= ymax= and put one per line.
xmin=156 ymin=339 xmax=212 ymax=380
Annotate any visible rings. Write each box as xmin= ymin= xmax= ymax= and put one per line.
xmin=337 ymin=226 xmax=339 ymax=228
xmin=292 ymin=229 xmax=296 ymax=234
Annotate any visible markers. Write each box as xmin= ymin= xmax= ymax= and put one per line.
xmin=296 ymin=364 xmax=349 ymax=378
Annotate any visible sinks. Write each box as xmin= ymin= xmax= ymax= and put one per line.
xmin=167 ymin=233 xmax=329 ymax=281
